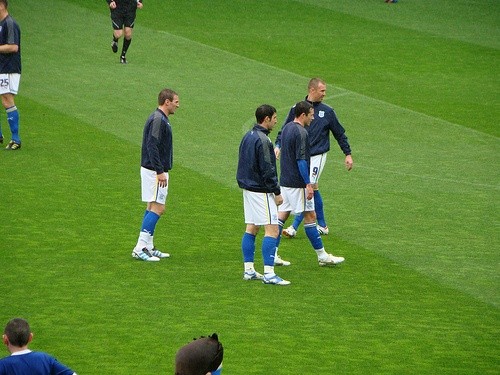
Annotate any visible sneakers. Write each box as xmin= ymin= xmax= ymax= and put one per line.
xmin=120 ymin=55 xmax=127 ymax=63
xmin=132 ymin=247 xmax=160 ymax=261
xmin=6 ymin=139 xmax=20 ymax=149
xmin=0 ymin=135 xmax=4 ymax=143
xmin=263 ymin=274 xmax=291 ymax=285
xmin=274 ymin=255 xmax=290 ymax=265
xmin=152 ymin=246 xmax=169 ymax=257
xmin=244 ymin=271 xmax=264 ymax=280
xmin=112 ymin=40 xmax=118 ymax=52
xmin=317 ymin=225 xmax=329 ymax=234
xmin=318 ymin=253 xmax=344 ymax=265
xmin=283 ymin=225 xmax=296 ymax=237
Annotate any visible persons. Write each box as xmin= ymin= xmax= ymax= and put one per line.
xmin=0 ymin=0 xmax=22 ymax=150
xmin=236 ymin=104 xmax=291 ymax=285
xmin=274 ymin=77 xmax=353 ymax=238
xmin=0 ymin=318 xmax=78 ymax=375
xmin=106 ymin=0 xmax=143 ymax=64
xmin=273 ymin=101 xmax=345 ymax=266
xmin=132 ymin=89 xmax=179 ymax=261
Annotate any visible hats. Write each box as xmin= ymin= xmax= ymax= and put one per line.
xmin=175 ymin=332 xmax=223 ymax=375
